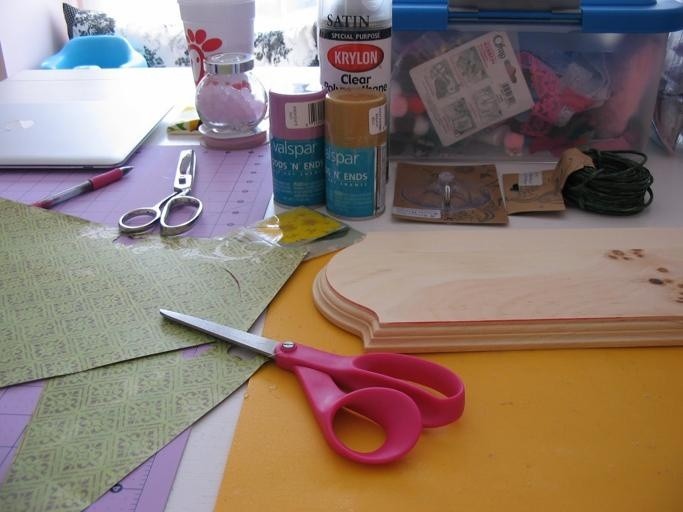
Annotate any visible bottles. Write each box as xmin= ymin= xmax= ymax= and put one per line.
xmin=325 ymin=87 xmax=386 ymax=221
xmin=192 ymin=49 xmax=270 ymax=140
xmin=269 ymin=82 xmax=326 ymax=211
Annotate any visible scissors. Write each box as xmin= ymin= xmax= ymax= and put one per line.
xmin=159 ymin=309 xmax=466 ymax=465
xmin=117 ymin=149 xmax=203 ymax=236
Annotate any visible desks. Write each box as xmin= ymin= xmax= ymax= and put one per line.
xmin=0 ymin=69 xmax=683 ymax=512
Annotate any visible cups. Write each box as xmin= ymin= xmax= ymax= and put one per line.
xmin=177 ymin=0 xmax=256 ymax=88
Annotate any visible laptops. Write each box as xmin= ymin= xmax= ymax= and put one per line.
xmin=0 ymin=101 xmax=175 ymax=168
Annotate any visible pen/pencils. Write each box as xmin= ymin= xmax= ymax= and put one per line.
xmin=388 ymin=79 xmax=526 ymax=151
xmin=30 ymin=165 xmax=134 ymax=208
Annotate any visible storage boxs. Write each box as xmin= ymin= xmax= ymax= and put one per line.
xmin=311 ymin=2 xmax=682 ymax=159
